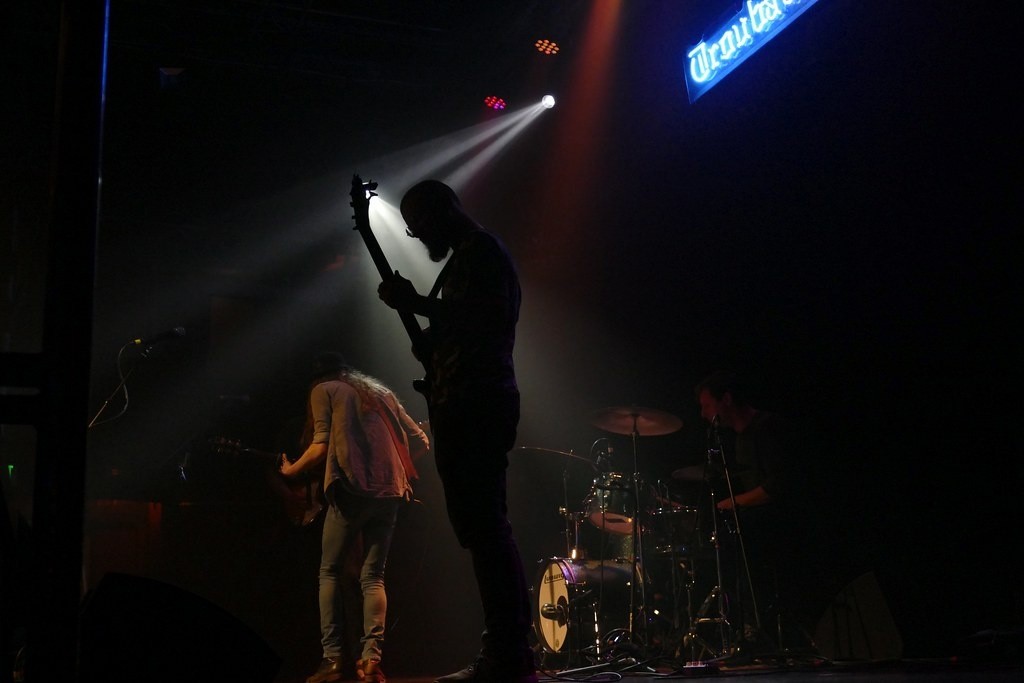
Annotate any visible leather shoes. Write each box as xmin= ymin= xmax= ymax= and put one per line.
xmin=306 ymin=655 xmax=344 ymax=683
xmin=433 ymin=648 xmax=538 ymax=683
xmin=362 ymin=658 xmax=382 ymax=683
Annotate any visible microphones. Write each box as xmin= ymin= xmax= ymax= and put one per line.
xmin=607 ymin=441 xmax=616 ymax=472
xmin=541 ymin=605 xmax=556 ymax=619
xmin=129 ymin=327 xmax=186 ymax=346
xmin=714 ymin=414 xmax=723 ymax=449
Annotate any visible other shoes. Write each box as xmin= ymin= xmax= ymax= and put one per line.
xmin=723 ymin=625 xmax=773 ymax=657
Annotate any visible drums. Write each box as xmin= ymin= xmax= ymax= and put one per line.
xmin=534 ymin=556 xmax=656 ymax=652
xmin=585 ymin=470 xmax=650 ymax=536
xmin=509 ymin=445 xmax=591 ymax=468
xmin=645 ymin=507 xmax=707 ymax=558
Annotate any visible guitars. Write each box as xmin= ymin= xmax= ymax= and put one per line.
xmin=205 ymin=433 xmax=327 ymax=476
xmin=349 ymin=175 xmax=437 ymax=390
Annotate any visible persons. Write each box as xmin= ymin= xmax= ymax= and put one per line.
xmin=677 ymin=368 xmax=815 ymax=650
xmin=378 ymin=181 xmax=537 ymax=683
xmin=278 ymin=351 xmax=430 ymax=683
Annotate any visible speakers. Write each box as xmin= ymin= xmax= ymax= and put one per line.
xmin=76 ymin=571 xmax=284 ymax=683
xmin=813 ymin=569 xmax=966 ymax=662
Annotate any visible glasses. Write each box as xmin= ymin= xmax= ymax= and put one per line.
xmin=406 ymin=228 xmax=416 ymax=237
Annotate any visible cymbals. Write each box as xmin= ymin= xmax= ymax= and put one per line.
xmin=670 ymin=463 xmax=753 ymax=481
xmin=588 ymin=406 xmax=684 ymax=437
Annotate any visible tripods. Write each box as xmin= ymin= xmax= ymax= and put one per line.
xmin=672 ymin=429 xmax=755 ymax=670
